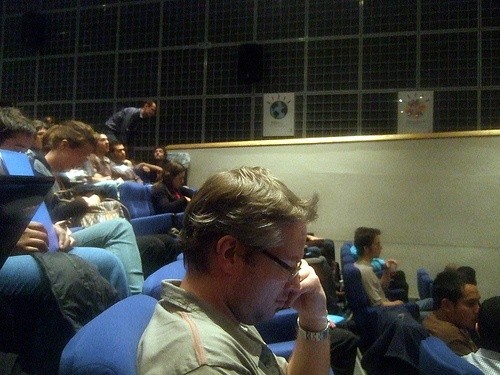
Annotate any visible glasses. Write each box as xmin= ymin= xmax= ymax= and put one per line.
xmin=256 ymin=245 xmax=302 ymax=278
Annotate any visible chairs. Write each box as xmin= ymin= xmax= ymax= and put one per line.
xmin=0 ymin=149 xmax=488 ymax=375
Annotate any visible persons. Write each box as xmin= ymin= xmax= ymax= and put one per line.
xmin=0 ymin=107 xmax=191 ymax=296
xmin=349 ymin=227 xmax=433 ymax=322
xmin=104 ymin=97 xmax=160 ymax=144
xmin=422 ymin=263 xmax=500 ymax=375
xmin=137 ymin=167 xmax=330 ymax=375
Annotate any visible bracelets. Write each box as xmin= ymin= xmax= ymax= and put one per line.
xmin=297 ymin=317 xmax=331 ymax=345
